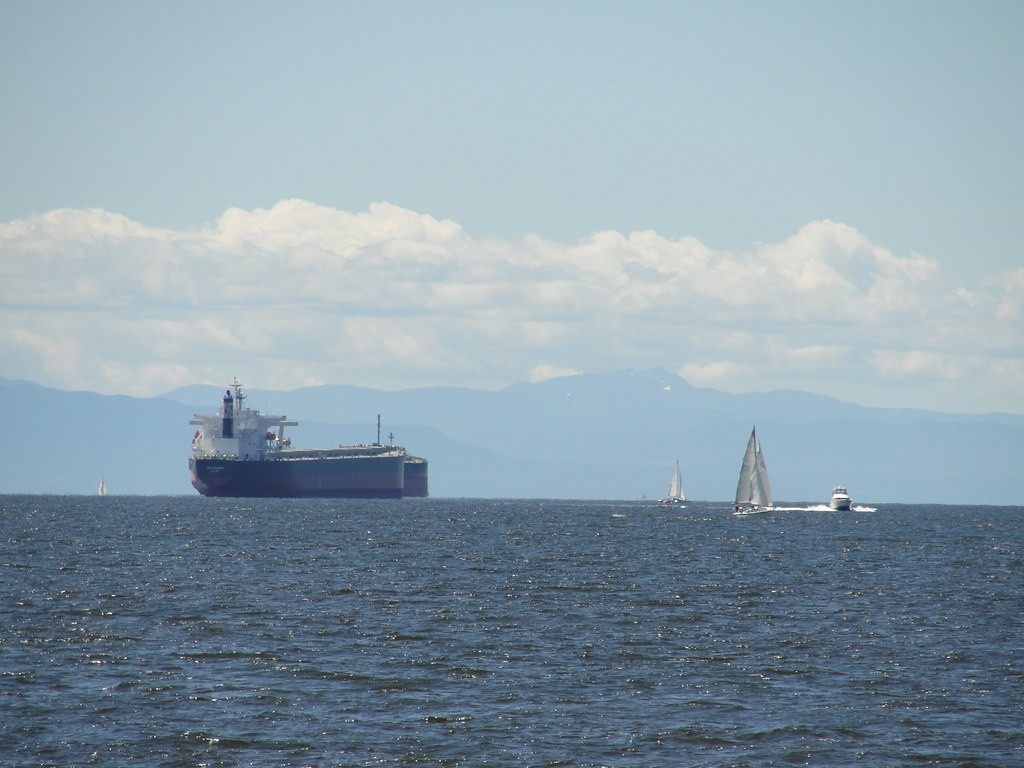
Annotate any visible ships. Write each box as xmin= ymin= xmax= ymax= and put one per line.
xmin=828 ymin=486 xmax=851 ymax=511
xmin=187 ymin=375 xmax=429 ymax=501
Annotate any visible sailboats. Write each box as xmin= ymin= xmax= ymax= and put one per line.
xmin=658 ymin=459 xmax=690 ymax=505
xmin=97 ymin=473 xmax=107 ymax=497
xmin=732 ymin=425 xmax=775 ymax=519
